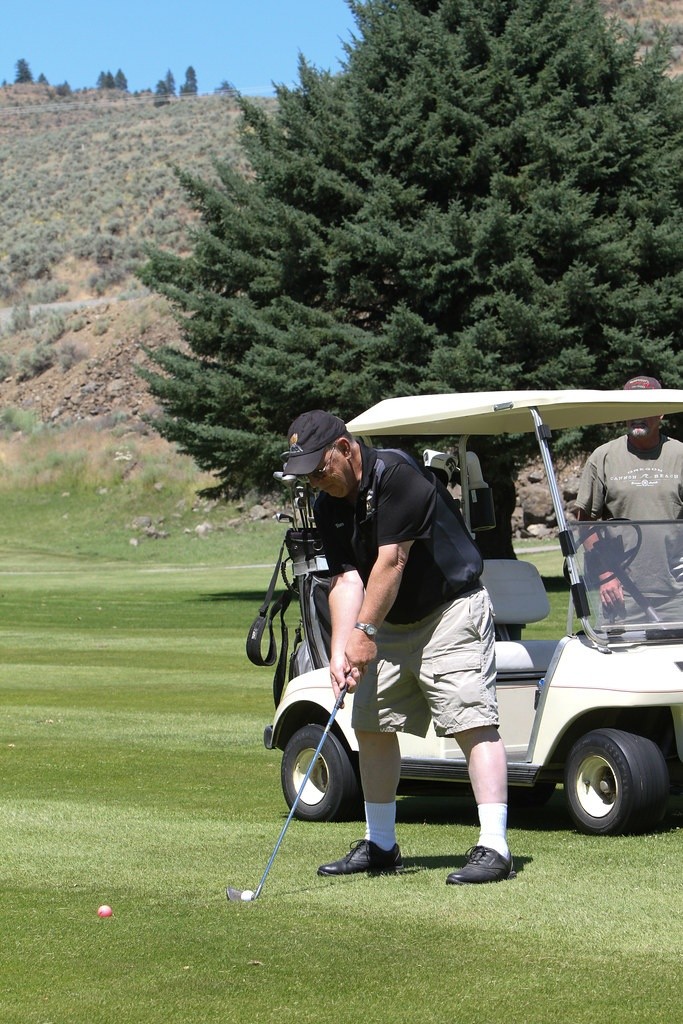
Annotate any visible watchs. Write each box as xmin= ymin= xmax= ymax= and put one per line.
xmin=353 ymin=622 xmax=379 ymax=639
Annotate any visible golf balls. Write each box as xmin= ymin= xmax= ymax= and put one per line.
xmin=240 ymin=890 xmax=255 ymax=902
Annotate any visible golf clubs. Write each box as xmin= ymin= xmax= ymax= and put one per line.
xmin=272 ymin=462 xmax=316 ymax=530
xmin=223 ymin=670 xmax=351 ymax=904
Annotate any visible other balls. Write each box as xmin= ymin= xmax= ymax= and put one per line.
xmin=98 ymin=905 xmax=112 ymax=918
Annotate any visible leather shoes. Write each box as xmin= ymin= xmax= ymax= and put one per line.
xmin=317 ymin=838 xmax=403 ymax=876
xmin=446 ymin=845 xmax=514 ymax=885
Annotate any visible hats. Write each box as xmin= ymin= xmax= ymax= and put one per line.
xmin=623 ymin=376 xmax=661 ymax=389
xmin=282 ymin=410 xmax=346 ymax=476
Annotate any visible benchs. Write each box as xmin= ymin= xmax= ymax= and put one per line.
xmin=479 ymin=560 xmax=561 ymax=673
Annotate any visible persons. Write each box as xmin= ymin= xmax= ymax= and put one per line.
xmin=281 ymin=410 xmax=515 ymax=888
xmin=573 ymin=375 xmax=683 ymax=621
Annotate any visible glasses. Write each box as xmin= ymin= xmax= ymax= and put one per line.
xmin=303 ymin=444 xmax=337 ymax=480
